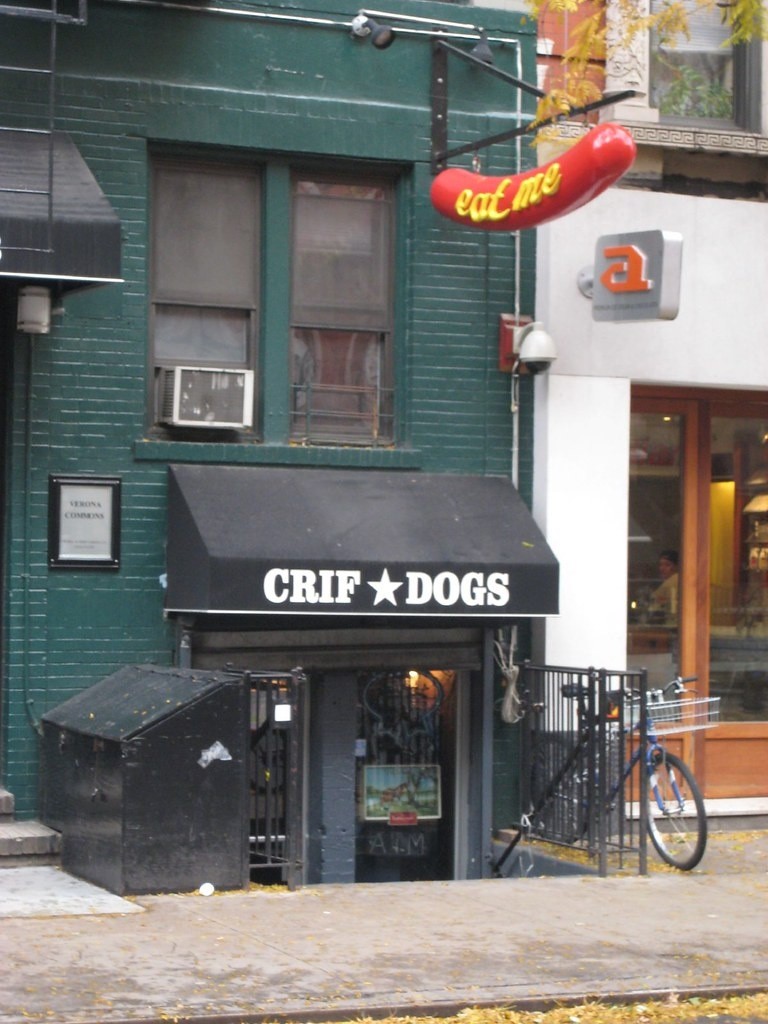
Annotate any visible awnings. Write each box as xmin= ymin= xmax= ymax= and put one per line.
xmin=0 ymin=128 xmax=126 ymax=295
xmin=162 ymin=463 xmax=560 ymax=618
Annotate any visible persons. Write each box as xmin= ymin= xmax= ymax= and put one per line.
xmin=649 ymin=550 xmax=678 ymax=624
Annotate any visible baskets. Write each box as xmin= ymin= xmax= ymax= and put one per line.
xmin=642 ymin=698 xmax=722 ymax=736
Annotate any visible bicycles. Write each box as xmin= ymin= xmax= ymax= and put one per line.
xmin=526 ymin=676 xmax=721 ymax=871
